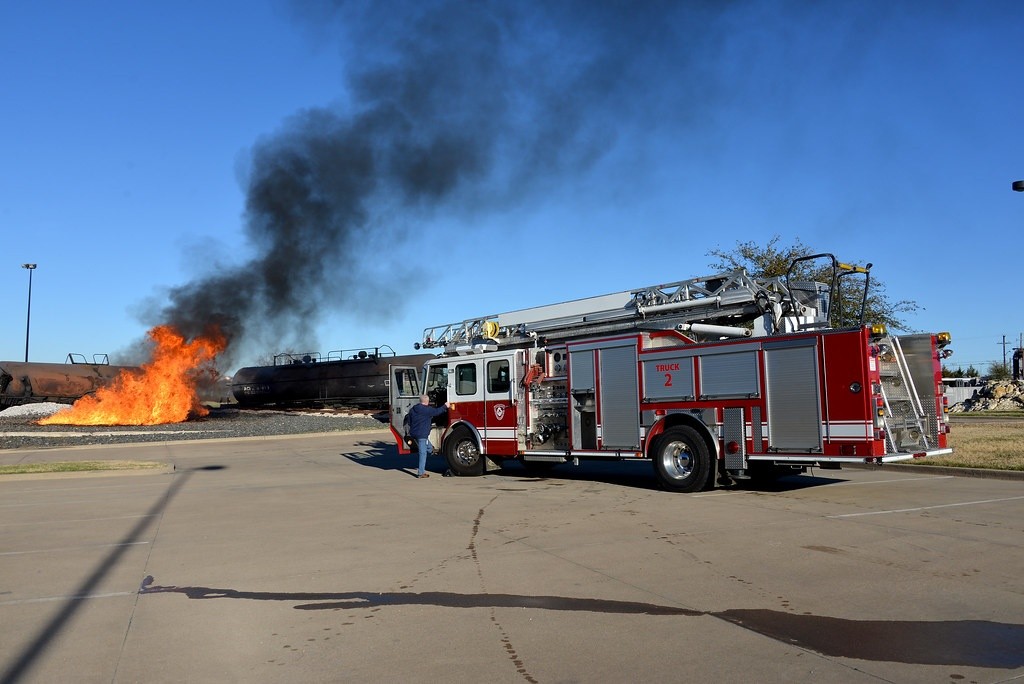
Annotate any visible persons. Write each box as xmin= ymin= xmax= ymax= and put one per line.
xmin=407 ymin=395 xmax=450 ymax=478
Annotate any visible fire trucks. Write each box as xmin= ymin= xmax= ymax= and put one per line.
xmin=386 ymin=252 xmax=954 ymax=492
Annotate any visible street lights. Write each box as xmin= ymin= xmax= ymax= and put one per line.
xmin=21 ymin=263 xmax=37 ymax=362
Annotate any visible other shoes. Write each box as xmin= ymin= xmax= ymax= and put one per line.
xmin=431 ymin=446 xmax=434 ymax=455
xmin=418 ymin=473 xmax=429 ymax=478
